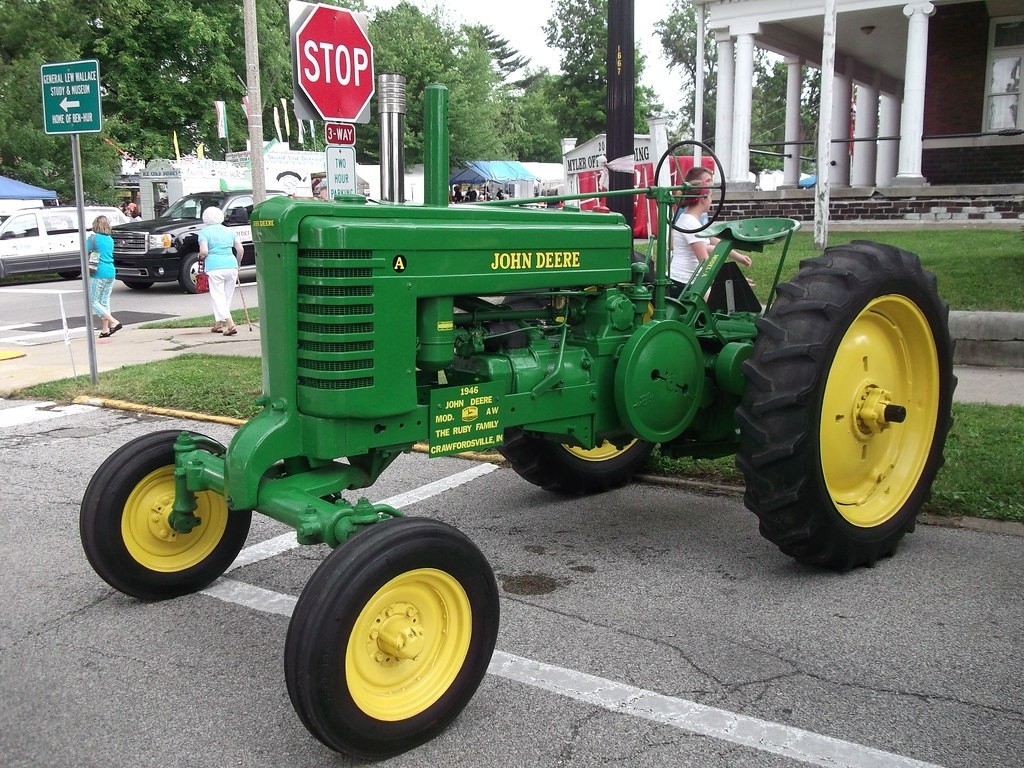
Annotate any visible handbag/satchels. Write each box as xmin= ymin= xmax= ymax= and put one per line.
xmin=195 ymin=255 xmax=210 ymax=294
xmin=88 ymin=234 xmax=100 ymax=276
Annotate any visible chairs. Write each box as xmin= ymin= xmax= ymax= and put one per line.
xmin=231 ymin=202 xmax=253 ymax=222
xmin=200 ymin=201 xmax=221 ymax=221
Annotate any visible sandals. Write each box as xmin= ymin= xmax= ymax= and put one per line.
xmin=210 ymin=325 xmax=224 ymax=333
xmin=222 ymin=325 xmax=237 ymax=336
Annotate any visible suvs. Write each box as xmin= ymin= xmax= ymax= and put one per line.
xmin=0 ymin=205 xmax=130 ymax=283
xmin=111 ymin=191 xmax=288 ymax=294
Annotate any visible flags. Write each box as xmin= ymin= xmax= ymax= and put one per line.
xmin=214 ymin=101 xmax=228 ymax=138
xmin=241 ymin=95 xmax=249 ymax=125
xmin=273 ymin=99 xmax=315 ymax=144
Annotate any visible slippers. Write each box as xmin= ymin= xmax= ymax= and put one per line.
xmin=107 ymin=323 xmax=123 ymax=336
xmin=99 ymin=331 xmax=111 ymax=338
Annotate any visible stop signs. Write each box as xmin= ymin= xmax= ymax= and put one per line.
xmin=296 ymin=4 xmax=375 ymax=123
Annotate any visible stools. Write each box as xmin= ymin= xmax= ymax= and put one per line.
xmin=694 ymin=215 xmax=802 ymax=246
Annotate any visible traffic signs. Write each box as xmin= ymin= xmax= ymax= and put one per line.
xmin=41 ymin=58 xmax=102 ymax=136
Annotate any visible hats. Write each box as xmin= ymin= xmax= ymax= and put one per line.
xmin=127 ymin=202 xmax=137 ymax=212
xmin=120 ymin=203 xmax=128 ymax=207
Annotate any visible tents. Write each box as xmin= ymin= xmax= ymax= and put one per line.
xmin=449 ymin=160 xmax=542 ymax=203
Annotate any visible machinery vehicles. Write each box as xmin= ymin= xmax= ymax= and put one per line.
xmin=79 ymin=138 xmax=960 ymax=764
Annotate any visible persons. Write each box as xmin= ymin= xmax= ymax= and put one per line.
xmin=86 ymin=215 xmax=122 ymax=337
xmin=496 ymin=190 xmax=502 ymax=199
xmin=198 ymin=206 xmax=245 ymax=336
xmin=452 ymin=186 xmax=478 ymax=202
xmin=124 ymin=202 xmax=142 ymax=221
xmin=666 ymin=166 xmax=756 ymax=302
